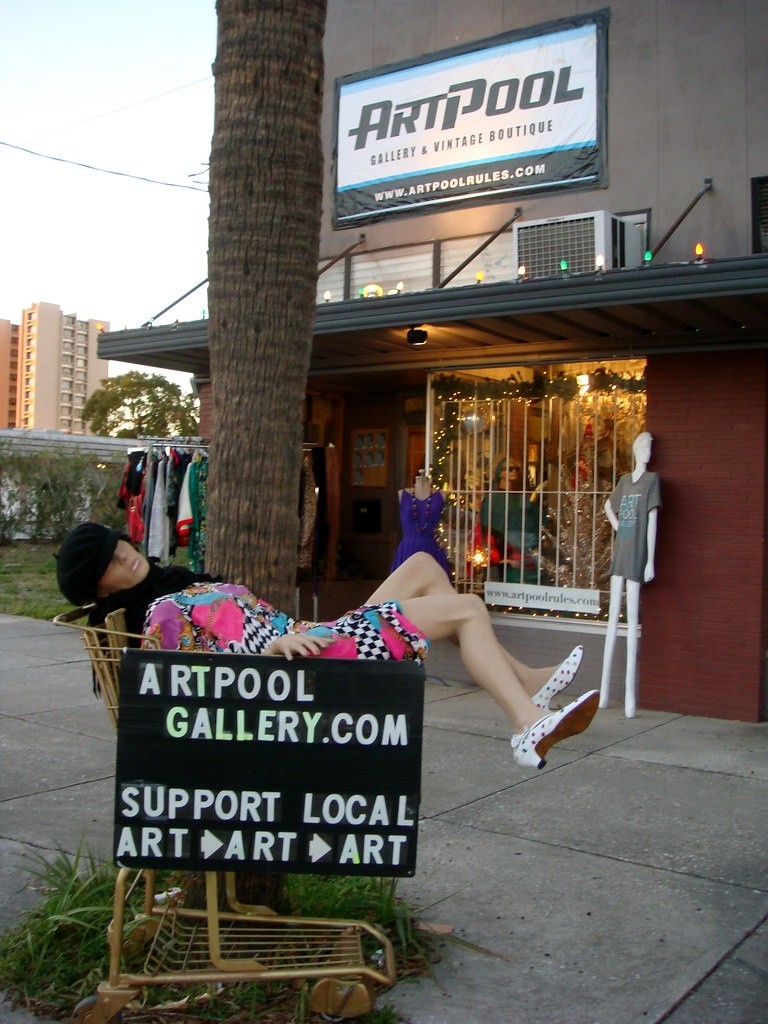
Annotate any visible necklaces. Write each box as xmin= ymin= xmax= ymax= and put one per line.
xmin=410 ymin=483 xmax=432 ymax=532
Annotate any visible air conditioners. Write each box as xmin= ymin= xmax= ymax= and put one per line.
xmin=513 ymin=210 xmax=643 ymax=280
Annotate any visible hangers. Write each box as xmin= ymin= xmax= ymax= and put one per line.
xmin=142 ymin=436 xmax=210 ymax=455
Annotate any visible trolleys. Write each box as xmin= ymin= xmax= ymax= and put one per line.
xmin=55 ymin=604 xmax=396 ymax=1024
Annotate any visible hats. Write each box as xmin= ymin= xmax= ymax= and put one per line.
xmin=52 ymin=522 xmax=120 ymax=606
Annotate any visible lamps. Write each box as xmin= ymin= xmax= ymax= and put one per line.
xmin=407 ymin=325 xmax=428 ymax=345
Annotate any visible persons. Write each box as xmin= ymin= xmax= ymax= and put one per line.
xmin=597 ymin=432 xmax=659 ymax=714
xmin=54 ymin=519 xmax=602 ymax=767
xmin=393 ymin=475 xmax=452 ymax=573
xmin=481 ymin=458 xmax=550 ymax=582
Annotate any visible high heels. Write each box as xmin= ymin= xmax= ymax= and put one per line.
xmin=528 ymin=645 xmax=584 ymax=714
xmin=510 ymin=690 xmax=601 ymax=770
